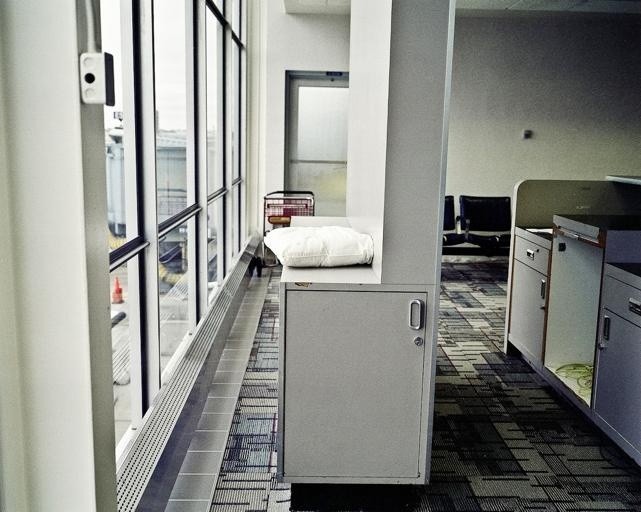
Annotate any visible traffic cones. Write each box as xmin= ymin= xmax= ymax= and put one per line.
xmin=111 ymin=276 xmax=122 ymax=304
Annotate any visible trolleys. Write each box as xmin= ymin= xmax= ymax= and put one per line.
xmin=255 ymin=190 xmax=314 ymax=277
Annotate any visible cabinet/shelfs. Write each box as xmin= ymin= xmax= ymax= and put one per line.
xmin=500 ymin=224 xmax=640 ymax=468
xmin=280 ymin=282 xmax=436 ymax=493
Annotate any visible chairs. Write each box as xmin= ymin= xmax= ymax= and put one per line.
xmin=442 ymin=190 xmax=519 ymax=276
